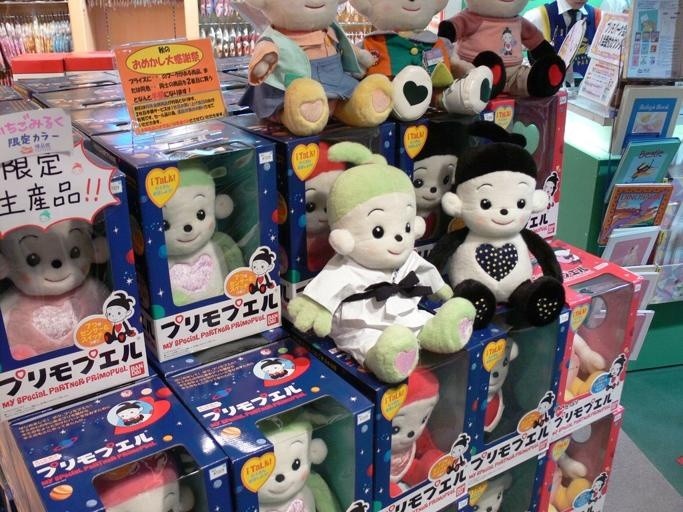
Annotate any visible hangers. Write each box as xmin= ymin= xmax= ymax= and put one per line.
xmin=0 ymin=0 xmax=374 ymax=38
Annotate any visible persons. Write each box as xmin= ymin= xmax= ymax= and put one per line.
xmin=522 ymin=0 xmax=604 ymax=87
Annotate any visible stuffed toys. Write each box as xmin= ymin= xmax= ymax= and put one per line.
xmin=163 ymin=142 xmax=607 ymax=511
xmin=230 ymin=1 xmax=566 ymax=136
xmin=94 ymin=451 xmax=194 ymax=511
xmin=2 ymin=219 xmax=111 ymax=360
xmin=0 ymin=0 xmax=606 ymax=512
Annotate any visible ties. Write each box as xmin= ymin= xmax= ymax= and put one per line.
xmin=567 ymin=10 xmax=578 ymax=33
